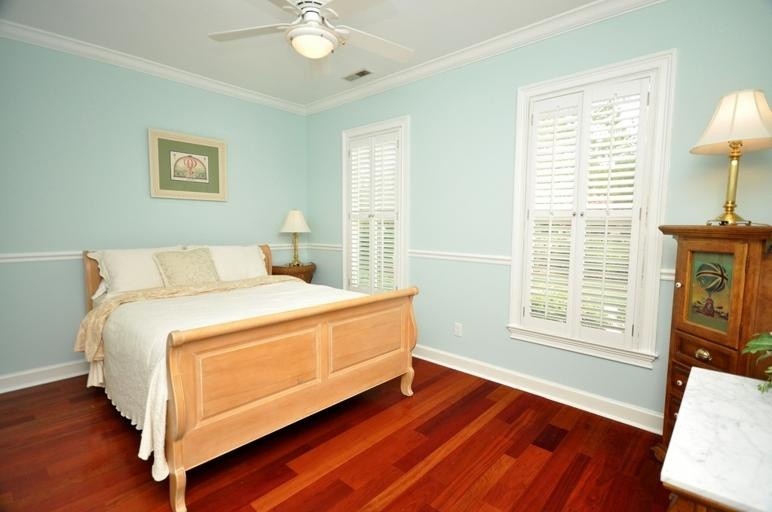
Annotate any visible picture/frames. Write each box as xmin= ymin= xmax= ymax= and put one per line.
xmin=148 ymin=128 xmax=228 ymax=202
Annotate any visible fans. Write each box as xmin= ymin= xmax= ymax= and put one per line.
xmin=207 ymin=0 xmax=415 ymax=65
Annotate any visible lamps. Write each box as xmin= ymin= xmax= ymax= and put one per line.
xmin=286 ymin=21 xmax=338 ymax=59
xmin=689 ymin=89 xmax=772 ymax=226
xmin=279 ymin=210 xmax=311 ymax=266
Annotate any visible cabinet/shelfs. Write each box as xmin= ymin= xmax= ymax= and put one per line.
xmin=648 ymin=225 xmax=772 ymax=461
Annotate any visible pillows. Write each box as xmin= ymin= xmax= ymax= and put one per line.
xmin=87 ymin=245 xmax=184 ymax=303
xmin=153 ymin=247 xmax=221 ymax=288
xmin=184 ymin=243 xmax=269 ymax=282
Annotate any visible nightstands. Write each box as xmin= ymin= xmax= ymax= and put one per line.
xmin=272 ymin=262 xmax=317 ymax=283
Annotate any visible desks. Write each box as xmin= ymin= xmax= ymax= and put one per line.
xmin=659 ymin=366 xmax=772 ymax=512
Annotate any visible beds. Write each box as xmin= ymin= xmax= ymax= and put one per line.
xmin=83 ymin=244 xmax=420 ymax=512
